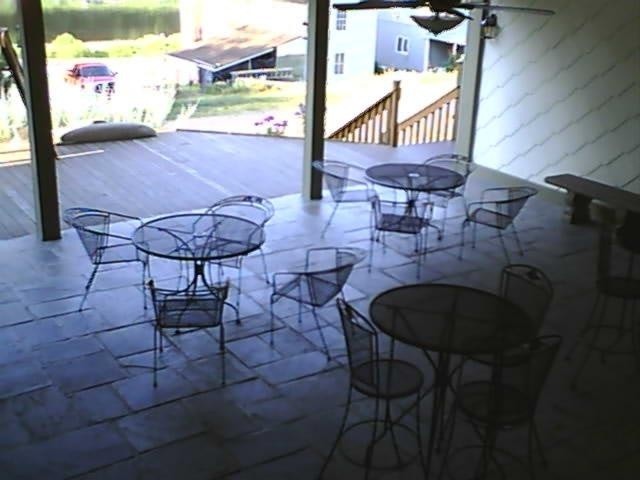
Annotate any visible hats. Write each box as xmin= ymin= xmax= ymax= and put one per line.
xmin=63 ymin=61 xmax=119 ymax=101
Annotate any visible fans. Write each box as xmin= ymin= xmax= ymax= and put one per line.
xmin=332 ymin=0 xmax=554 ymax=20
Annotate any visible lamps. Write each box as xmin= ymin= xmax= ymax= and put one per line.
xmin=410 ymin=15 xmax=465 ymax=37
xmin=480 ymin=14 xmax=497 ymax=39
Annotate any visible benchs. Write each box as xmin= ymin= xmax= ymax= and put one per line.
xmin=546 ymin=170 xmax=637 ymax=220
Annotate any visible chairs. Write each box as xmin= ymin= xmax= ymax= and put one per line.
xmin=269 ymin=246 xmax=367 ymax=362
xmin=437 ymin=335 xmax=563 ymax=477
xmin=312 ymin=160 xmax=381 ymax=240
xmin=192 ymin=194 xmax=275 ymax=314
xmin=367 ymin=197 xmax=435 ymax=280
xmin=418 ymin=156 xmax=477 ymax=230
xmin=474 ymin=264 xmax=553 ymax=390
xmin=458 ymin=187 xmax=540 ymax=262
xmin=152 ymin=280 xmax=229 ymax=386
xmin=321 ymin=298 xmax=425 ymax=478
xmin=568 ymin=221 xmax=637 ymax=364
xmin=64 ymin=206 xmax=154 ymax=310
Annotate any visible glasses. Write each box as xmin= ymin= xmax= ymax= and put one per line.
xmin=410 ymin=15 xmax=465 ymax=37
xmin=480 ymin=14 xmax=497 ymax=39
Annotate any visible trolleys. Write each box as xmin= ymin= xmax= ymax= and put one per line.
xmin=332 ymin=0 xmax=554 ymax=20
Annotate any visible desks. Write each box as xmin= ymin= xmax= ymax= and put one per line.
xmin=370 ymin=281 xmax=534 ymax=450
xmin=366 ymin=163 xmax=464 ymax=249
xmin=134 ymin=214 xmax=266 ymax=325
